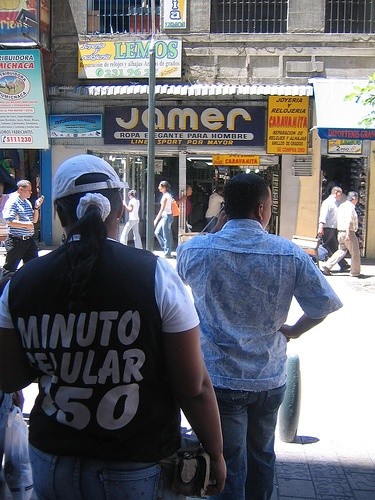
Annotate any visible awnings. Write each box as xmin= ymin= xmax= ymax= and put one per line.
xmin=76 ymin=86 xmax=313 ymax=97
xmin=309 ymin=77 xmax=375 ymax=130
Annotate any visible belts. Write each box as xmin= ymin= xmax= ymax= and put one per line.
xmin=9 ymin=235 xmax=31 ymax=240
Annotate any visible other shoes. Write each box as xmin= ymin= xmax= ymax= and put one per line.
xmin=352 ymin=273 xmax=363 ymax=277
xmin=321 ymin=265 xmax=332 ymax=276
xmin=341 ymin=265 xmax=351 ymax=271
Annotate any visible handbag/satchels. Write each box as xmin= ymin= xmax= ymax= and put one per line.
xmin=317 ymin=235 xmax=328 ymax=261
xmin=4 ymin=404 xmax=34 ymax=500
xmin=160 ymin=437 xmax=211 ymax=498
xmin=172 ymin=198 xmax=180 ymax=216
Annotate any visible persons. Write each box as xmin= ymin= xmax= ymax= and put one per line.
xmin=205 ymin=187 xmax=224 ymax=231
xmin=154 ymin=181 xmax=174 ymax=257
xmin=172 ymin=185 xmax=192 ymax=239
xmin=2 ymin=179 xmax=44 ymax=270
xmin=321 ymin=192 xmax=360 ymax=277
xmin=120 ymin=189 xmax=143 ymax=249
xmin=174 ymin=172 xmax=344 ymax=500
xmin=0 ymin=153 xmax=225 ymax=500
xmin=318 ymin=186 xmax=350 ymax=272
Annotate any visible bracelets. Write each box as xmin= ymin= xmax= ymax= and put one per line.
xmin=34 ymin=207 xmax=39 ymax=210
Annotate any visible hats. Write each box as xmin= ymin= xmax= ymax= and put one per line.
xmin=53 ymin=155 xmax=129 ymax=200
xmin=0 ymin=164 xmax=19 ymax=194
